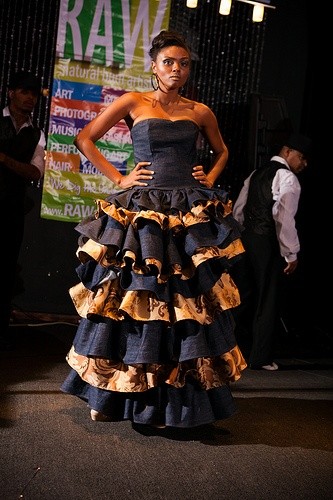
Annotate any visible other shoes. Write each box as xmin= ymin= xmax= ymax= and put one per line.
xmin=261 ymin=361 xmax=279 ymax=370
xmin=90 ymin=408 xmax=112 ymax=420
xmin=10 ymin=306 xmax=26 ymax=320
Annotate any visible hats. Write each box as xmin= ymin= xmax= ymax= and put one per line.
xmin=8 ymin=71 xmax=43 ymax=97
xmin=285 ymin=133 xmax=316 ymax=161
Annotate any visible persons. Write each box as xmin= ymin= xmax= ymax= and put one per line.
xmin=0 ymin=72 xmax=46 ymax=322
xmin=233 ymin=133 xmax=310 ymax=371
xmin=60 ymin=31 xmax=248 ymax=428
